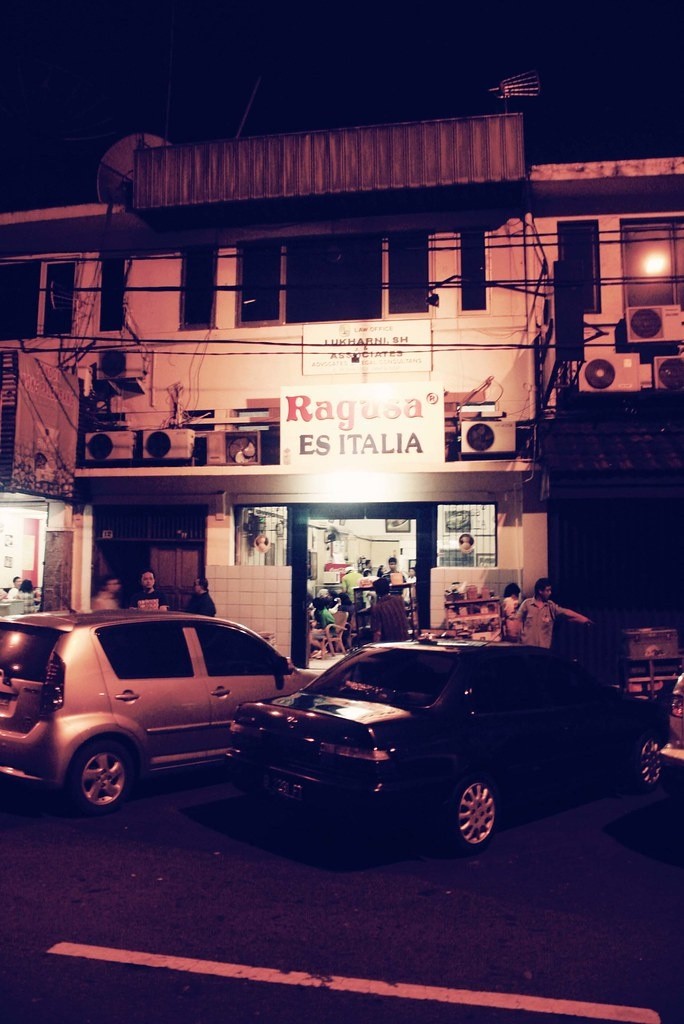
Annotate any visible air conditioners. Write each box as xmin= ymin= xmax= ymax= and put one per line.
xmin=460 ymin=421 xmax=516 ymax=454
xmin=653 ymin=355 xmax=684 ymax=391
xmin=96 ymin=346 xmax=145 ymax=382
xmin=207 ymin=429 xmax=262 ymax=466
xmin=578 ymin=353 xmax=642 ymax=393
xmin=142 ymin=429 xmax=196 ymax=459
xmin=625 ymin=304 xmax=684 ymax=343
xmin=85 ymin=431 xmax=136 ymax=460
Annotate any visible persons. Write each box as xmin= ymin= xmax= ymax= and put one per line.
xmin=93 ymin=576 xmax=122 ymax=609
xmin=128 ymin=568 xmax=171 ymax=611
xmin=8 ymin=576 xmax=22 ymax=599
xmin=381 ymin=557 xmax=408 ymax=584
xmin=503 ymin=583 xmax=525 ymax=642
xmin=306 ymin=588 xmax=355 ymax=626
xmin=34 ymin=587 xmax=42 ymax=605
xmin=363 ymin=560 xmax=373 ymax=578
xmin=341 ymin=565 xmax=368 ymax=609
xmin=369 ymin=577 xmax=411 ymax=645
xmin=17 ymin=580 xmax=36 ymax=614
xmin=516 ymin=576 xmax=595 ymax=652
xmin=377 ymin=565 xmax=384 ymax=578
xmin=406 ymin=567 xmax=417 ymax=598
xmin=185 ymin=576 xmax=218 ymax=618
xmin=309 ymin=596 xmax=337 ymax=658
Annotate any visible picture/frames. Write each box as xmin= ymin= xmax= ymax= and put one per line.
xmin=445 ymin=510 xmax=471 ymax=533
xmin=409 ymin=559 xmax=417 ymax=569
xmin=329 ymin=519 xmax=334 ymax=523
xmin=339 ymin=520 xmax=345 ymax=526
xmin=385 ymin=519 xmax=411 ymax=533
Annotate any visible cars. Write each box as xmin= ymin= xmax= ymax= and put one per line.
xmin=226 ymin=640 xmax=669 ymax=851
xmin=0 ymin=584 xmax=324 ymax=813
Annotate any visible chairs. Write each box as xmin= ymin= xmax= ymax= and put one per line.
xmin=322 ymin=611 xmax=349 ymax=658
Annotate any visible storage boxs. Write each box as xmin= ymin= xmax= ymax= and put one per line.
xmin=623 ymin=625 xmax=677 ymax=659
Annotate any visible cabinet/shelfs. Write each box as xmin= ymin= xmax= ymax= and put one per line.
xmin=354 ymin=581 xmax=418 ymax=646
xmin=443 ymin=597 xmax=502 ymax=642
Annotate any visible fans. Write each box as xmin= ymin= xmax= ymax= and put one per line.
xmin=324 ymin=526 xmax=341 ymax=552
